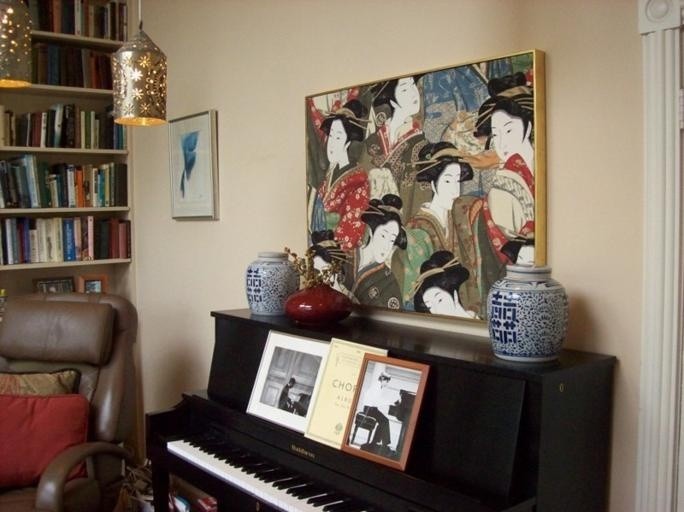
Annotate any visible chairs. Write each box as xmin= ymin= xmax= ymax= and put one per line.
xmin=0 ymin=295 xmax=138 ymax=512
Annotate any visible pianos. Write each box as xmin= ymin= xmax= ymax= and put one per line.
xmin=146 ymin=310 xmax=616 ymax=508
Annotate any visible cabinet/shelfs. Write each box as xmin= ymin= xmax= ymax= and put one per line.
xmin=0 ymin=0 xmax=141 ymax=296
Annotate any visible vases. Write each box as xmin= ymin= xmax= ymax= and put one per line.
xmin=243 ymin=252 xmax=300 ymax=318
xmin=486 ymin=263 xmax=570 ymax=362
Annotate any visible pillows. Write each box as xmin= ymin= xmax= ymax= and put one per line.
xmin=0 ymin=393 xmax=92 ymax=485
xmin=0 ymin=367 xmax=81 ymax=397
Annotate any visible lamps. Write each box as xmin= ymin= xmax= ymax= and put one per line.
xmin=112 ymin=0 xmax=169 ymax=125
xmin=0 ymin=0 xmax=34 ymax=88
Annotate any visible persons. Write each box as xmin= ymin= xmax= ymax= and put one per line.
xmin=315 ymin=98 xmax=372 ymax=251
xmin=349 ymin=193 xmax=408 ymax=312
xmin=501 ymin=220 xmax=536 ymax=266
xmin=475 ymin=73 xmax=535 ymax=264
xmin=278 ymin=377 xmax=298 ymax=412
xmin=364 ymin=73 xmax=433 ymax=226
xmin=397 ymin=141 xmax=484 ymax=317
xmin=405 ymin=250 xmax=482 ymax=321
xmin=310 ymin=229 xmax=362 ymax=306
xmin=363 ymin=370 xmax=400 ymax=452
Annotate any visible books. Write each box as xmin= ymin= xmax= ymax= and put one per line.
xmin=0 ymin=1 xmax=133 ymax=270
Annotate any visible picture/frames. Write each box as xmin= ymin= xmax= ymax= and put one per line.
xmin=339 ymin=353 xmax=430 ymax=470
xmin=78 ymin=274 xmax=109 ymax=293
xmin=32 ymin=275 xmax=76 ymax=295
xmin=304 ymin=48 xmax=548 ymax=322
xmin=166 ymin=109 xmax=218 ymax=221
xmin=303 ymin=337 xmax=389 ymax=450
xmin=245 ymin=329 xmax=330 ymax=434
